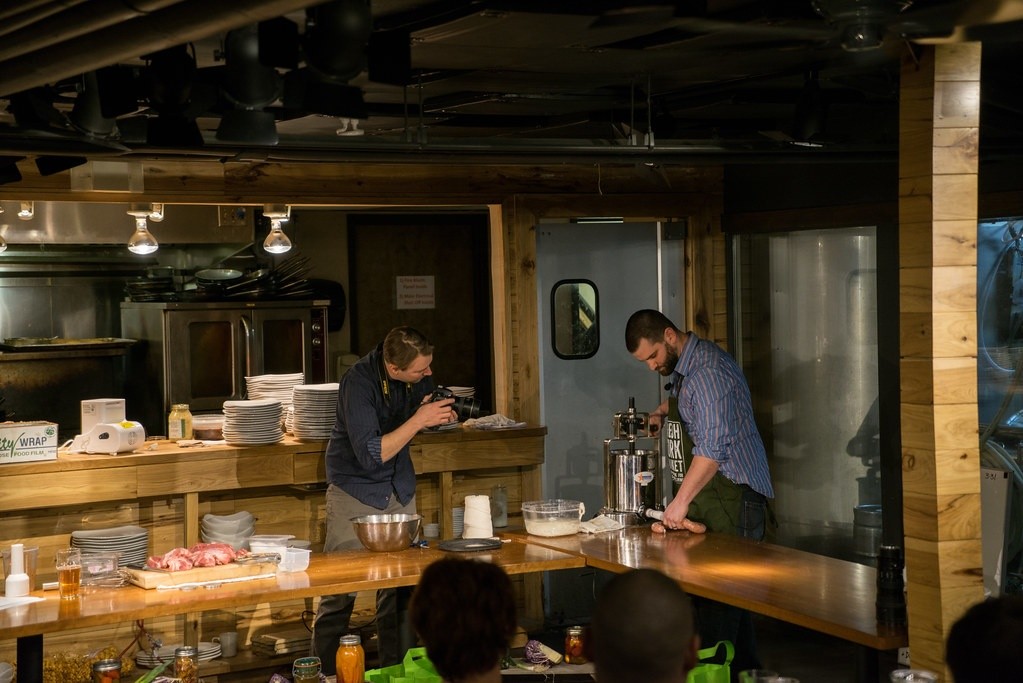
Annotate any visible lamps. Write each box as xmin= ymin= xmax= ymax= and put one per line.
xmin=17 ymin=202 xmax=35 ymax=220
xmin=125 ymin=203 xmax=159 ymax=254
xmin=261 ymin=203 xmax=292 ymax=253
xmin=64 ymin=0 xmax=373 ymax=148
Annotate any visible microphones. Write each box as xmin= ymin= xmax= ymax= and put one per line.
xmin=664 ymin=382 xmax=672 ymax=391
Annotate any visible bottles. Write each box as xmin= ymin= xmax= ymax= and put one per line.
xmin=174 ymin=646 xmax=199 ymax=683
xmin=491 ymin=483 xmax=508 ymax=528
xmin=93 ymin=659 xmax=121 ymax=683
xmin=565 ymin=625 xmax=586 ymax=664
xmin=169 ymin=404 xmax=192 ymax=443
xmin=336 ymin=634 xmax=364 ymax=683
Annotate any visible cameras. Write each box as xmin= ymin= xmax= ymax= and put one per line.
xmin=429 ymin=385 xmax=483 ymax=418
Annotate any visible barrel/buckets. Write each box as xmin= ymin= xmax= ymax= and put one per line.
xmin=850 ymin=503 xmax=883 ymax=559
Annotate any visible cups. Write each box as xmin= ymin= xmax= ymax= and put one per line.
xmin=212 ymin=632 xmax=238 ymax=657
xmin=56 ymin=547 xmax=81 ymax=599
xmin=0 ymin=545 xmax=39 ymax=592
xmin=739 ymin=669 xmax=800 ymax=683
xmin=889 ymin=669 xmax=941 ymax=683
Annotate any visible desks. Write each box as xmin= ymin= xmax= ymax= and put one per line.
xmin=0 ymin=534 xmax=584 ymax=683
xmin=516 ymin=510 xmax=906 ymax=683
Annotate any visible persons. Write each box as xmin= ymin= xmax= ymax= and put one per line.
xmin=943 ymin=595 xmax=1023 ymax=683
xmin=310 ymin=325 xmax=458 ymax=683
xmin=411 ymin=556 xmax=519 ymax=683
xmin=582 ymin=568 xmax=701 ymax=683
xmin=625 ymin=309 xmax=776 ymax=540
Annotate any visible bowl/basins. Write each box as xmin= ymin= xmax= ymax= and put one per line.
xmin=350 ymin=514 xmax=424 ymax=552
xmin=520 ymin=498 xmax=580 ymax=536
xmin=201 ymin=510 xmax=255 ymax=553
xmin=191 ymin=414 xmax=224 ymax=440
xmin=248 ymin=534 xmax=295 ymax=553
xmin=288 ymin=540 xmax=312 ymax=550
xmin=194 ymin=268 xmax=244 ymax=292
xmin=438 ymin=507 xmax=464 ymax=538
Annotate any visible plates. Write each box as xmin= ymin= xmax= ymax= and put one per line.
xmin=136 ymin=642 xmax=222 ymax=669
xmin=71 ymin=525 xmax=148 ymax=568
xmin=221 ymin=373 xmax=340 ymax=447
xmin=419 ymin=386 xmax=476 ymax=433
xmin=422 ymin=523 xmax=440 ymax=538
